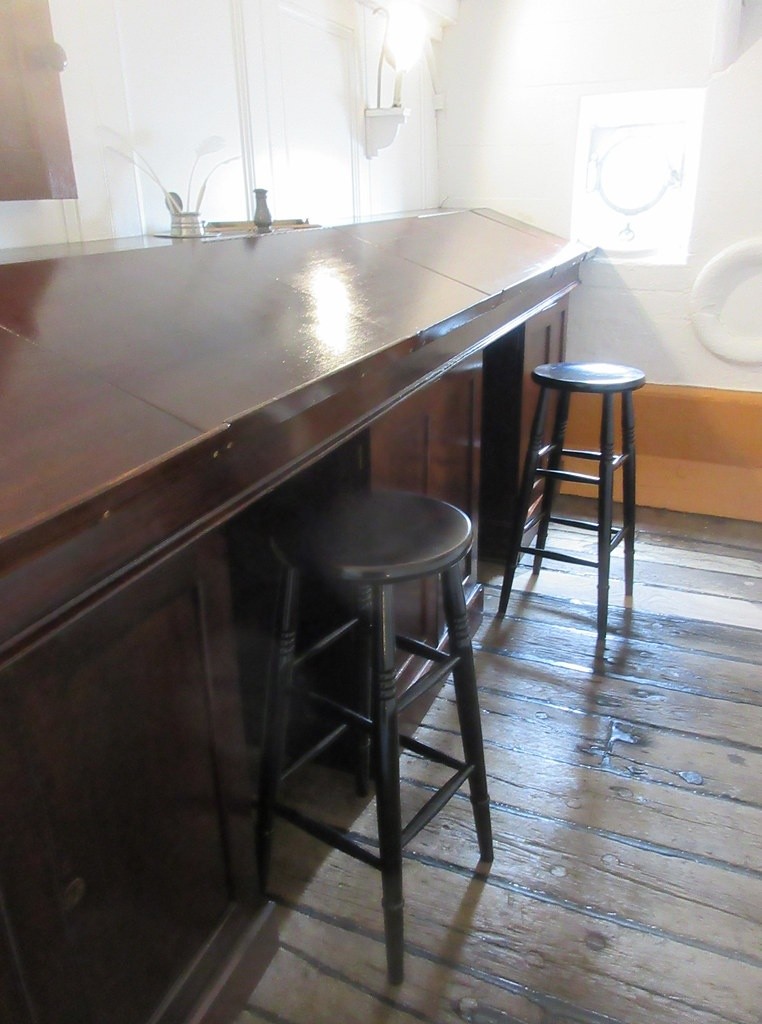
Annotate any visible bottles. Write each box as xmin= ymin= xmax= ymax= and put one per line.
xmin=253 ymin=188 xmax=273 ymax=233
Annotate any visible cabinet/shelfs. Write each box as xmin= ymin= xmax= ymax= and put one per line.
xmin=0 ymin=0 xmax=79 ymax=203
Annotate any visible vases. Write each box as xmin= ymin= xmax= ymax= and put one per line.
xmin=252 ymin=188 xmax=273 ymax=233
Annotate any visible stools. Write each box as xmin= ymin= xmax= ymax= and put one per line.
xmin=246 ymin=484 xmax=498 ymax=986
xmin=491 ymin=358 xmax=651 ymax=641
